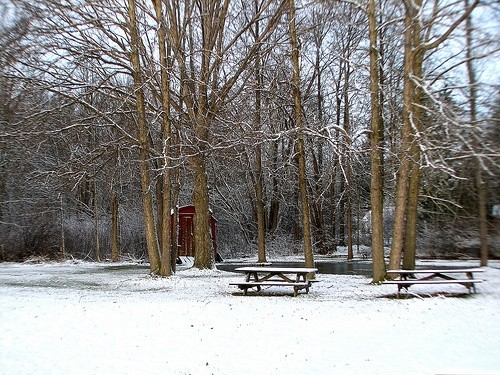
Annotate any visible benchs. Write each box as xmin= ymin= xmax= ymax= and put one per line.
xmin=247 ymin=279 xmax=318 ymax=288
xmin=229 ymin=281 xmax=311 ymax=297
xmin=379 ymin=280 xmax=481 ymax=298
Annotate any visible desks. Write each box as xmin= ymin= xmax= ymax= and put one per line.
xmin=386 ymin=269 xmax=484 ymax=295
xmin=235 ymin=267 xmax=318 ymax=297
